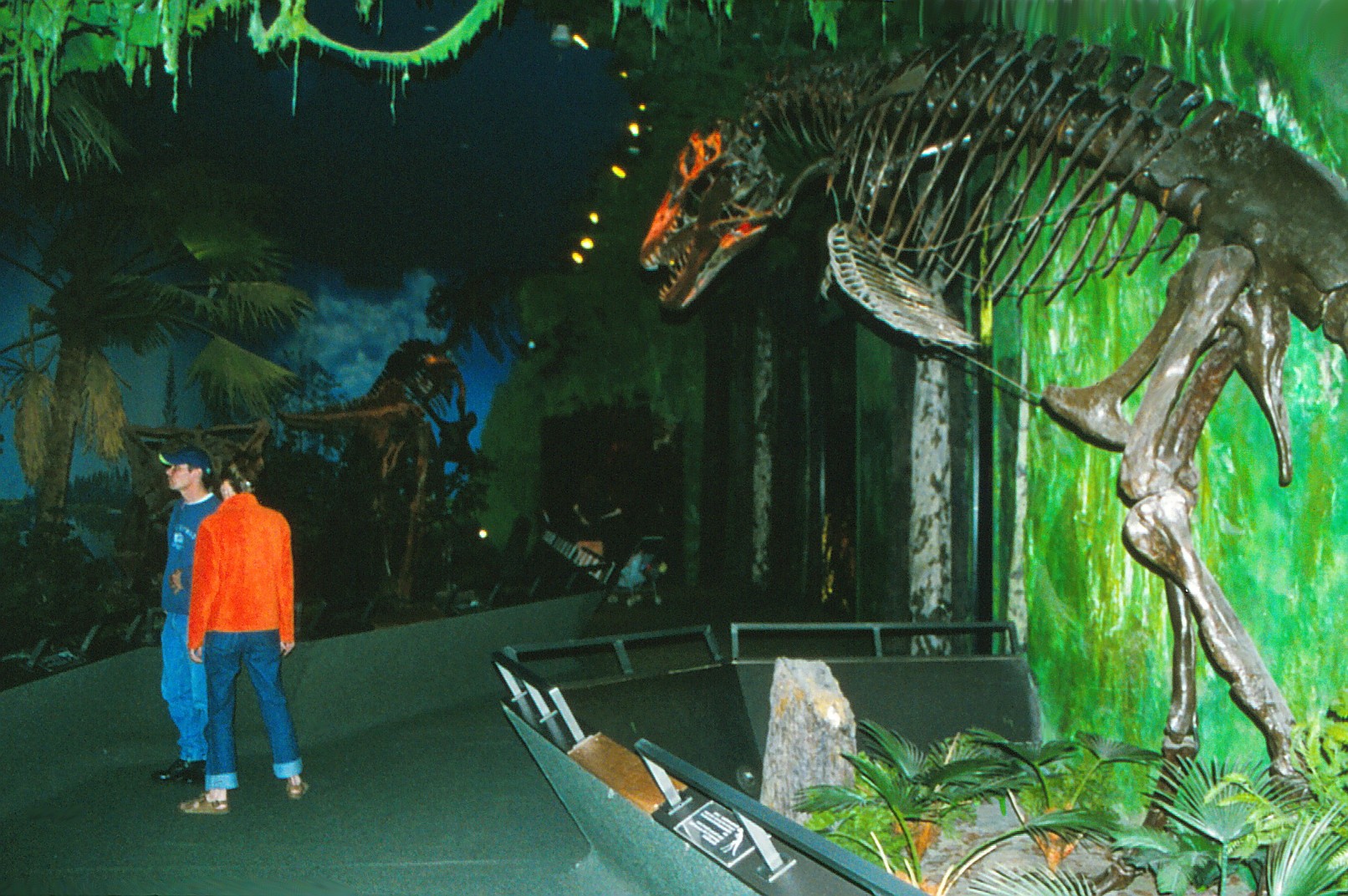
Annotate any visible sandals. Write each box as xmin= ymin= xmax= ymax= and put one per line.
xmin=179 ymin=792 xmax=229 ymax=814
xmin=286 ymin=779 xmax=310 ymax=800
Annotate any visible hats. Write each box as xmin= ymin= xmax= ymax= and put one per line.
xmin=158 ymin=445 xmax=212 ymax=469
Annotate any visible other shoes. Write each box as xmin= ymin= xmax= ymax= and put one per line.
xmin=151 ymin=757 xmax=206 ymax=784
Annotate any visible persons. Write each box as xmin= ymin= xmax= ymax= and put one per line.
xmin=149 ymin=448 xmax=223 ymax=787
xmin=180 ymin=451 xmax=310 ymax=817
